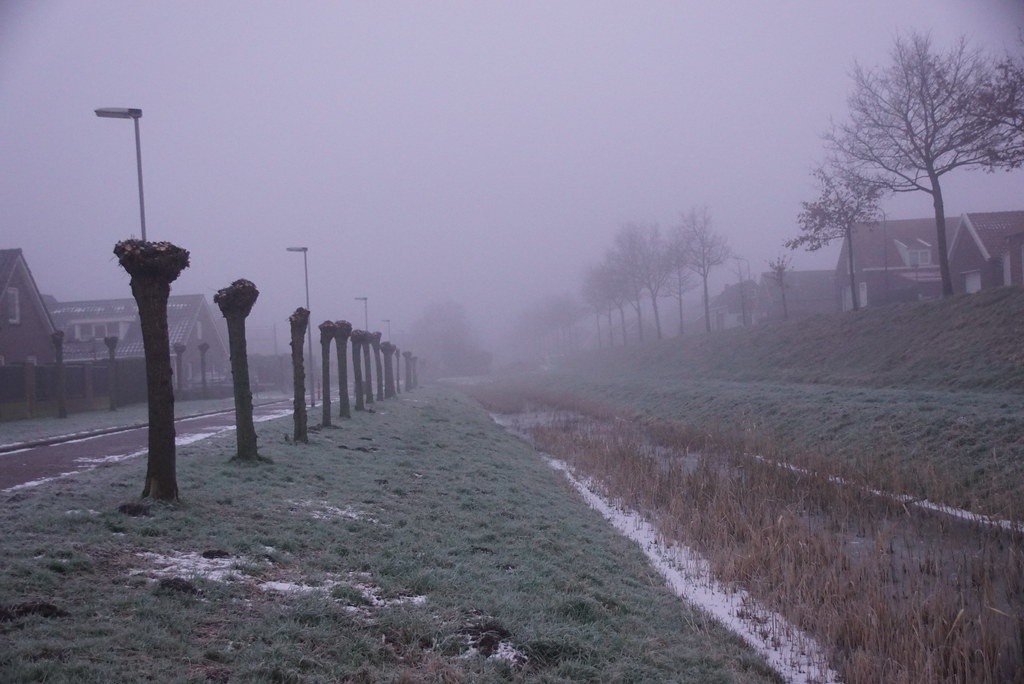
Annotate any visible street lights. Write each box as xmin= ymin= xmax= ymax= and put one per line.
xmin=94 ymin=106 xmax=147 ymax=247
xmin=287 ymin=246 xmax=315 ymax=410
xmin=354 ymin=296 xmax=368 ymax=330
xmin=380 ymin=319 xmax=391 ymax=342
